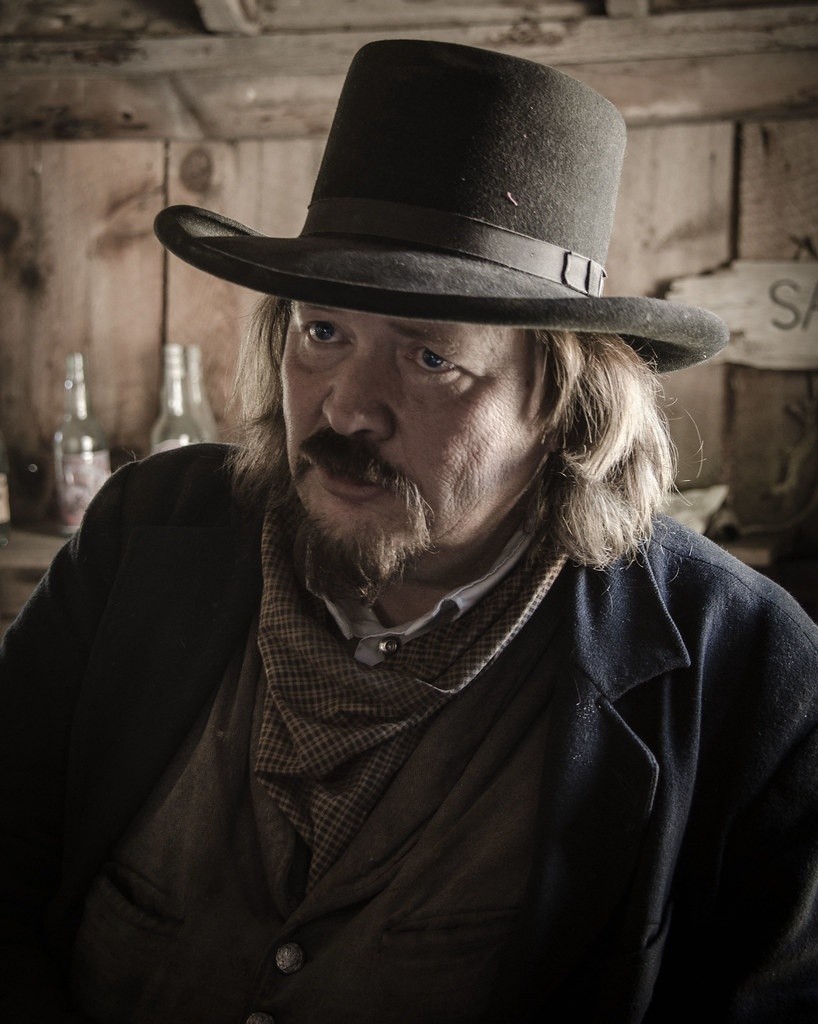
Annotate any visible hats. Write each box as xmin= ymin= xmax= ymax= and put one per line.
xmin=152 ymin=38 xmax=731 ymax=373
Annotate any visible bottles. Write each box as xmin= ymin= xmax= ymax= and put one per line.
xmin=0 ymin=432 xmax=11 ymax=549
xmin=53 ymin=353 xmax=112 ymax=538
xmin=150 ymin=343 xmax=222 ymax=456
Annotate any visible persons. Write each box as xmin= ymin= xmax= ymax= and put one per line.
xmin=0 ymin=37 xmax=818 ymax=1024
